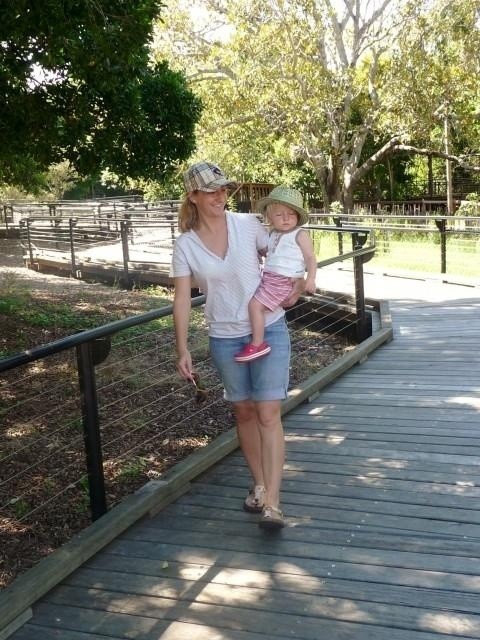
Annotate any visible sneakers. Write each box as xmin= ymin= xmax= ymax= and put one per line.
xmin=234 ymin=341 xmax=272 ymax=363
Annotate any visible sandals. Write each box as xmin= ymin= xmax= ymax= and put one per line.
xmin=243 ymin=484 xmax=266 ymax=513
xmin=258 ymin=505 xmax=284 ymax=529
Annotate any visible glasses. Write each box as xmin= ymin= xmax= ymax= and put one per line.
xmin=187 ymin=372 xmax=209 ymax=408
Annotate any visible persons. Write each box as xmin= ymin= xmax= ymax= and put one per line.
xmin=233 ymin=185 xmax=318 ymax=364
xmin=167 ymin=160 xmax=306 ymax=531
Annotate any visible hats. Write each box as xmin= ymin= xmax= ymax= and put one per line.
xmin=183 ymin=161 xmax=237 ymax=193
xmin=257 ymin=187 xmax=309 ymax=226
